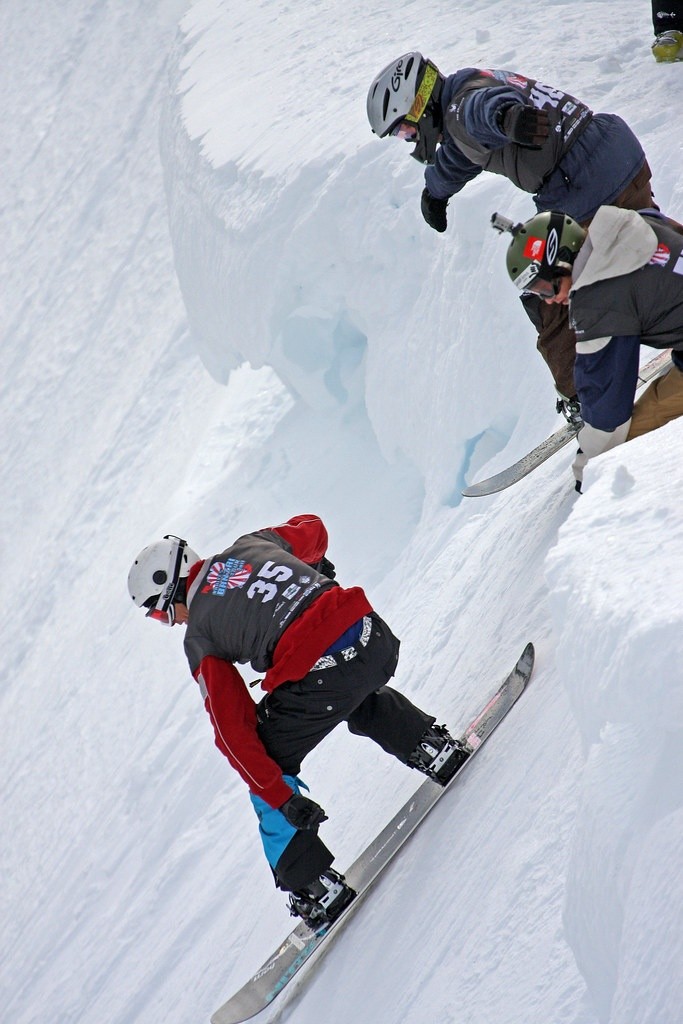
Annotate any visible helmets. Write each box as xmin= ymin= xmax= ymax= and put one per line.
xmin=490 ymin=210 xmax=588 ymax=292
xmin=128 ymin=538 xmax=202 ymax=608
xmin=365 ymin=51 xmax=446 ymax=167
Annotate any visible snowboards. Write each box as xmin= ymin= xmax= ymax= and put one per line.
xmin=459 ymin=347 xmax=675 ymax=499
xmin=208 ymin=643 xmax=536 ymax=1024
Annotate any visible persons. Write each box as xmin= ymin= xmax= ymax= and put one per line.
xmin=367 ymin=51 xmax=660 ymax=423
xmin=651 ymin=0 xmax=683 ymax=60
xmin=506 ymin=205 xmax=683 ymax=494
xmin=128 ymin=514 xmax=464 ymax=930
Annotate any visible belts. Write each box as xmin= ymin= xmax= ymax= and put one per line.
xmin=309 ymin=615 xmax=372 ymax=673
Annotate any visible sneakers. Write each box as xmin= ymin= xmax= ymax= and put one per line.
xmin=285 ymin=868 xmax=357 ymax=937
xmin=555 ymin=397 xmax=583 ymax=433
xmin=405 ymin=724 xmax=468 ymax=787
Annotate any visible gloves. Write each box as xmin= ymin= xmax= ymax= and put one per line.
xmin=420 ymin=187 xmax=450 ymax=233
xmin=279 ymin=793 xmax=329 ymax=831
xmin=503 ymin=103 xmax=551 ymax=146
xmin=307 ymin=556 xmax=336 ymax=580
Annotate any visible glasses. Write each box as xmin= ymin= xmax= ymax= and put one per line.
xmin=145 ymin=603 xmax=177 ymax=627
xmin=389 ymin=116 xmax=420 ymax=143
xmin=522 ymin=269 xmax=563 ymax=301
